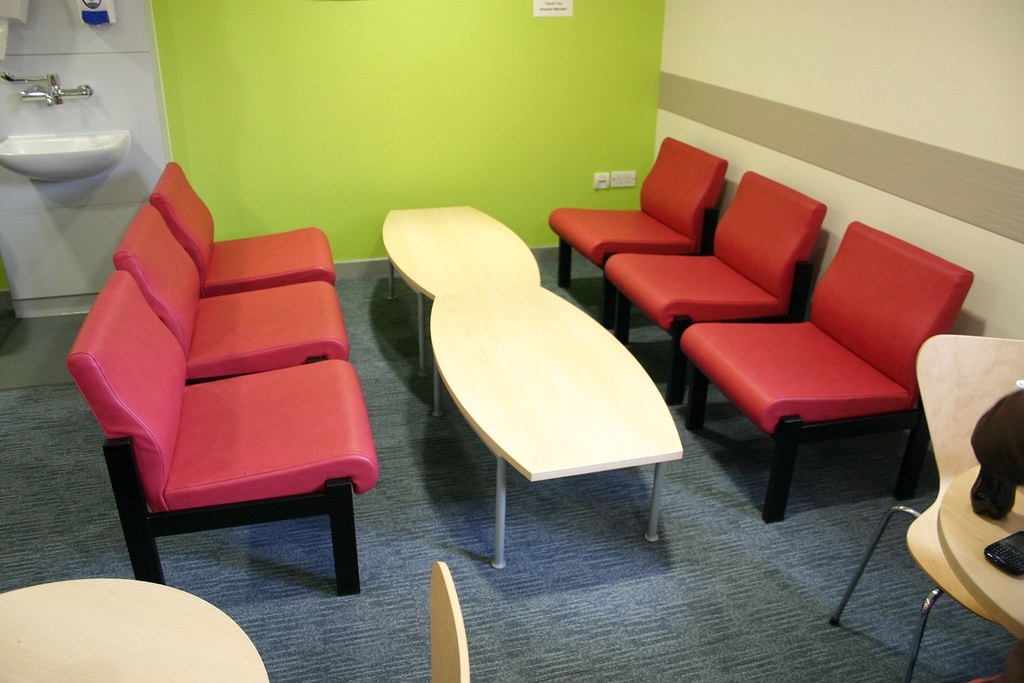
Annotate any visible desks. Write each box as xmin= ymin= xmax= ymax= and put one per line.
xmin=381 ymin=204 xmax=542 ymax=377
xmin=431 ymin=286 xmax=685 ymax=569
xmin=937 ymin=466 xmax=1024 ymax=639
xmin=0 ymin=579 xmax=270 ymax=683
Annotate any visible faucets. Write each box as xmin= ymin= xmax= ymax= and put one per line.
xmin=1 ymin=73 xmax=62 ymax=109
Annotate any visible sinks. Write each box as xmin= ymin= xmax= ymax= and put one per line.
xmin=1 ymin=130 xmax=132 ymax=183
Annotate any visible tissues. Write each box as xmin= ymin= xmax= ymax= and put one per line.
xmin=0 ymin=0 xmax=29 ymax=61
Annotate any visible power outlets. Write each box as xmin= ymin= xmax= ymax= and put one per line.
xmin=611 ymin=170 xmax=636 ymax=188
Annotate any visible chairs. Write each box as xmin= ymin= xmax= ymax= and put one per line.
xmin=148 ymin=161 xmax=336 ymax=299
xmin=604 ymin=171 xmax=829 ymax=406
xmin=547 ymin=135 xmax=729 ymax=329
xmin=66 ymin=270 xmax=380 ymax=596
xmin=432 ymin=561 xmax=487 ymax=683
xmin=682 ymin=221 xmax=976 ymax=524
xmin=831 ymin=334 xmax=1024 ymax=683
xmin=113 ymin=204 xmax=350 ymax=385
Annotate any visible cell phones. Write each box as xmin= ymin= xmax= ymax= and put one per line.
xmin=983 ymin=531 xmax=1024 ymax=576
